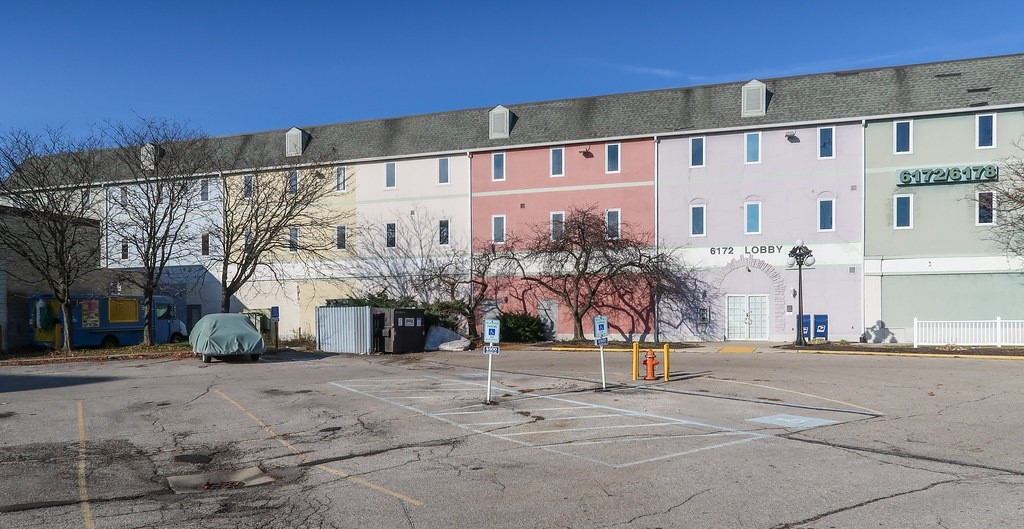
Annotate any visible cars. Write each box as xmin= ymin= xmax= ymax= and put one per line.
xmin=189 ymin=313 xmax=265 ymax=363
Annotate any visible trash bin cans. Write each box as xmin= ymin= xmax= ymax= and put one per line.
xmin=796 ymin=314 xmax=810 ymax=341
xmin=814 ymin=314 xmax=828 ymax=340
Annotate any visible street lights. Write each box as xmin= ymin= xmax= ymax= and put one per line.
xmin=786 ymin=239 xmax=815 ymax=346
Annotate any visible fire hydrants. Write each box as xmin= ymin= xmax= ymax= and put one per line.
xmin=642 ymin=349 xmax=659 ymax=380
